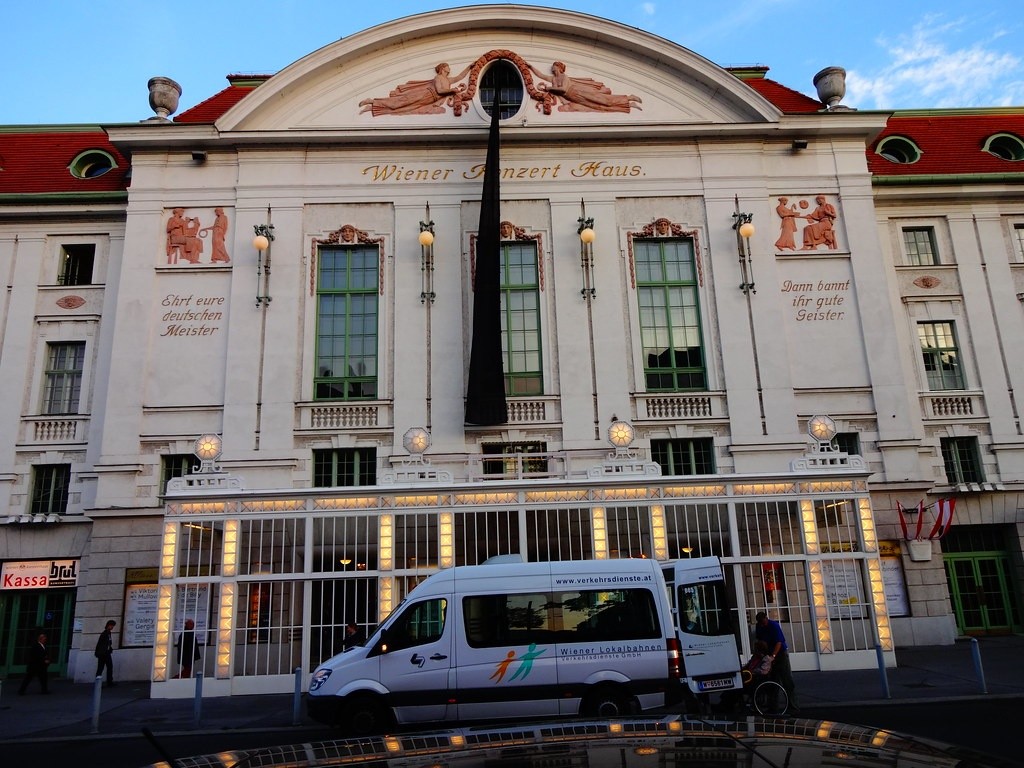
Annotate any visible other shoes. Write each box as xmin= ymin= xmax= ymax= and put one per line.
xmin=792 ymin=713 xmax=800 ymax=717
xmin=107 ymin=683 xmax=118 ymax=687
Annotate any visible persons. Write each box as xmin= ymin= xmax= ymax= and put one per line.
xmin=18 ymin=633 xmax=50 ymax=696
xmin=742 ymin=640 xmax=772 ymax=679
xmin=358 ymin=62 xmax=476 ymax=117
xmin=171 ymin=620 xmax=201 ymax=679
xmin=202 ymin=207 xmax=231 ymax=262
xmin=749 ymin=612 xmax=800 ymax=713
xmin=344 ymin=622 xmax=362 ymax=650
xmin=773 ymin=196 xmax=800 ymax=251
xmin=525 ymin=60 xmax=642 ymax=114
xmin=165 ymin=207 xmax=204 ymax=264
xmin=94 ymin=620 xmax=117 ymax=688
xmin=799 ymin=195 xmax=837 ymax=251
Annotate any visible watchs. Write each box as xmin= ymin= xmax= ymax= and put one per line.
xmin=772 ymin=654 xmax=776 ymax=658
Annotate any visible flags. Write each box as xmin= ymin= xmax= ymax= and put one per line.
xmin=896 ymin=495 xmax=956 ymax=542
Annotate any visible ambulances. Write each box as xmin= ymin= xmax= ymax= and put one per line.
xmin=306 ymin=554 xmax=743 ymax=732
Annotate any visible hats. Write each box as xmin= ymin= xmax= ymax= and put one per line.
xmin=185 ymin=620 xmax=194 ymax=630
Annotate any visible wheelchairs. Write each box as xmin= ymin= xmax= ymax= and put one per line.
xmin=748 ymin=658 xmax=789 ymax=716
